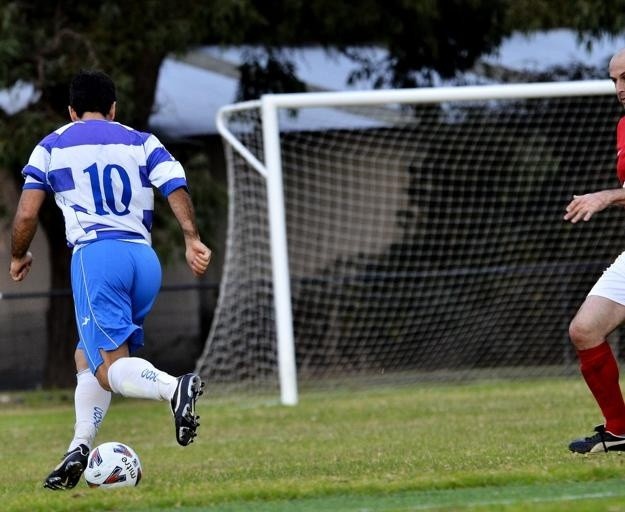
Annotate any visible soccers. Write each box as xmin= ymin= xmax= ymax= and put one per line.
xmin=85 ymin=442 xmax=142 ymax=489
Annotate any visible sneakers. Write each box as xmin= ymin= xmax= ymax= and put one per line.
xmin=171 ymin=374 xmax=205 ymax=447
xmin=569 ymin=425 xmax=625 ymax=455
xmin=42 ymin=444 xmax=89 ymax=491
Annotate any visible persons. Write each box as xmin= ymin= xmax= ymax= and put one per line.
xmin=565 ymin=47 xmax=624 ymax=457
xmin=8 ymin=70 xmax=213 ymax=488
